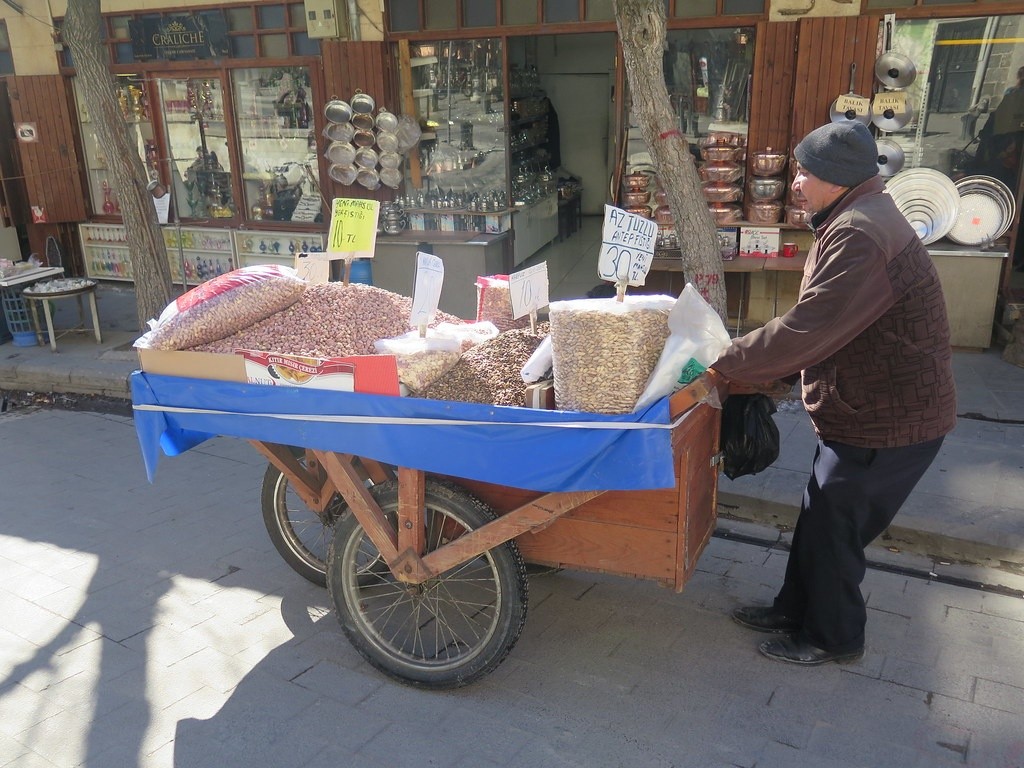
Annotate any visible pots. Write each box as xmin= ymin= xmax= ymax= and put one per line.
xmin=830 ymin=62 xmax=873 ymax=128
xmin=874 ymin=138 xmax=904 ymax=177
xmin=945 ymin=173 xmax=1016 ymax=247
xmin=873 ymin=21 xmax=916 ymax=89
xmin=619 ymin=131 xmax=812 ymax=228
xmin=882 ymin=168 xmax=961 ymax=245
xmin=870 ymin=90 xmax=913 ymax=133
xmin=322 ymin=40 xmax=580 ymax=196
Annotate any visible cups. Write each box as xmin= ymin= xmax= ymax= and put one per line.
xmin=394 ymin=190 xmax=508 ymax=212
xmin=783 ymin=242 xmax=798 ymax=257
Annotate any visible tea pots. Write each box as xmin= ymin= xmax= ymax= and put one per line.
xmin=375 ymin=200 xmax=407 ymax=236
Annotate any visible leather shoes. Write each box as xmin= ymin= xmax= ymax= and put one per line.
xmin=759 ymin=632 xmax=866 ymax=665
xmin=732 ymin=604 xmax=804 ymax=634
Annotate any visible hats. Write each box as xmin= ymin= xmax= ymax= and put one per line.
xmin=795 ymin=121 xmax=879 ymax=187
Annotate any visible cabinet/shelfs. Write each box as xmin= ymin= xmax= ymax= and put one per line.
xmin=78 ymin=224 xmax=327 ymax=285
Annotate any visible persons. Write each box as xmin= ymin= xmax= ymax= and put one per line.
xmin=709 ymin=115 xmax=956 ymax=666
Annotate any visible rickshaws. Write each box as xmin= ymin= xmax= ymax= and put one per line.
xmin=126 ymin=339 xmax=738 ymax=694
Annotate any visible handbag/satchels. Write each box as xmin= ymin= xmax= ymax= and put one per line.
xmin=719 ymin=393 xmax=779 ymax=482
